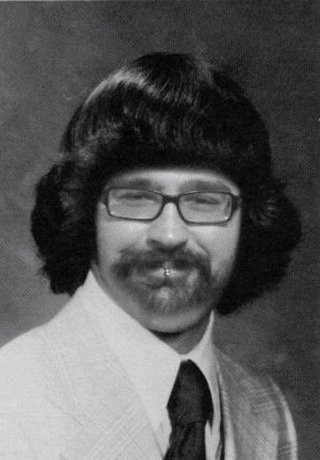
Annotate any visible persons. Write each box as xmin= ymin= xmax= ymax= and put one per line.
xmin=0 ymin=52 xmax=303 ymax=460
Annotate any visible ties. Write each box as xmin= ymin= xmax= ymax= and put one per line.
xmin=163 ymin=359 xmax=214 ymax=460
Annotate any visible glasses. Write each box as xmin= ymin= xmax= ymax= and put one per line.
xmin=94 ymin=187 xmax=242 ymax=225
